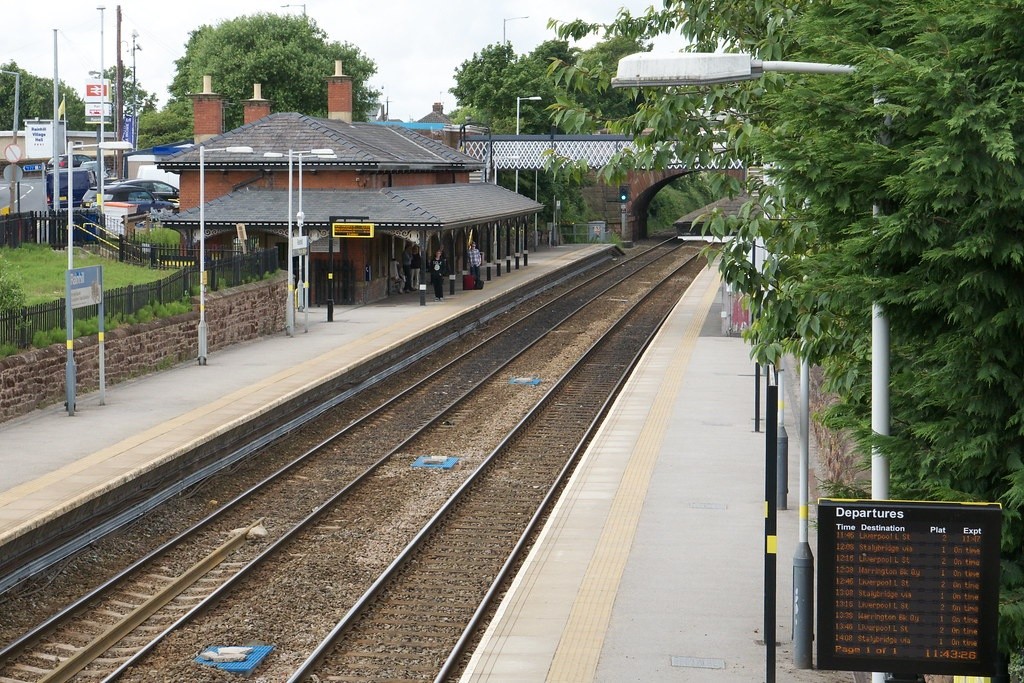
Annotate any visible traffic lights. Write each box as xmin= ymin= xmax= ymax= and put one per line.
xmin=618 ymin=185 xmax=629 ymax=203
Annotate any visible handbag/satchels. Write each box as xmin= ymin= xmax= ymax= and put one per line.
xmin=474 ymin=279 xmax=484 ymax=290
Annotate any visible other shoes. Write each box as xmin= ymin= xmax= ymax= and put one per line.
xmin=435 ymin=297 xmax=439 ymax=302
xmin=405 ymin=289 xmax=411 ymax=293
xmin=440 ymin=298 xmax=443 ymax=302
xmin=409 ymin=287 xmax=417 ymax=292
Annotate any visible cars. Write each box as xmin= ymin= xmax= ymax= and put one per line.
xmin=45 ymin=153 xmax=181 ymax=227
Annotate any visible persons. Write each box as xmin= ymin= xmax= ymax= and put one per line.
xmin=403 ymin=245 xmax=421 ymax=292
xmin=467 ymin=243 xmax=481 ymax=280
xmin=431 ymin=246 xmax=447 ymax=301
xmin=390 ymin=259 xmax=403 ymax=293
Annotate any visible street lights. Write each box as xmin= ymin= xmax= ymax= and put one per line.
xmin=263 ymin=152 xmax=337 ymax=313
xmin=287 ymin=147 xmax=334 ymax=336
xmin=67 ymin=142 xmax=134 ymax=349
xmin=502 ymin=15 xmax=530 ymax=47
xmin=198 ymin=146 xmax=253 ymax=367
xmin=515 ymin=96 xmax=542 ymax=195
xmin=0 ymin=69 xmax=19 ymax=213
xmin=608 ymin=50 xmax=889 ymax=683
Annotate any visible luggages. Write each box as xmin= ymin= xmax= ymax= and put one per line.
xmin=463 ymin=274 xmax=474 ymax=290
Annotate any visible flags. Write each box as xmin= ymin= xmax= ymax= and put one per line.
xmin=58 ymin=100 xmax=64 ymax=120
